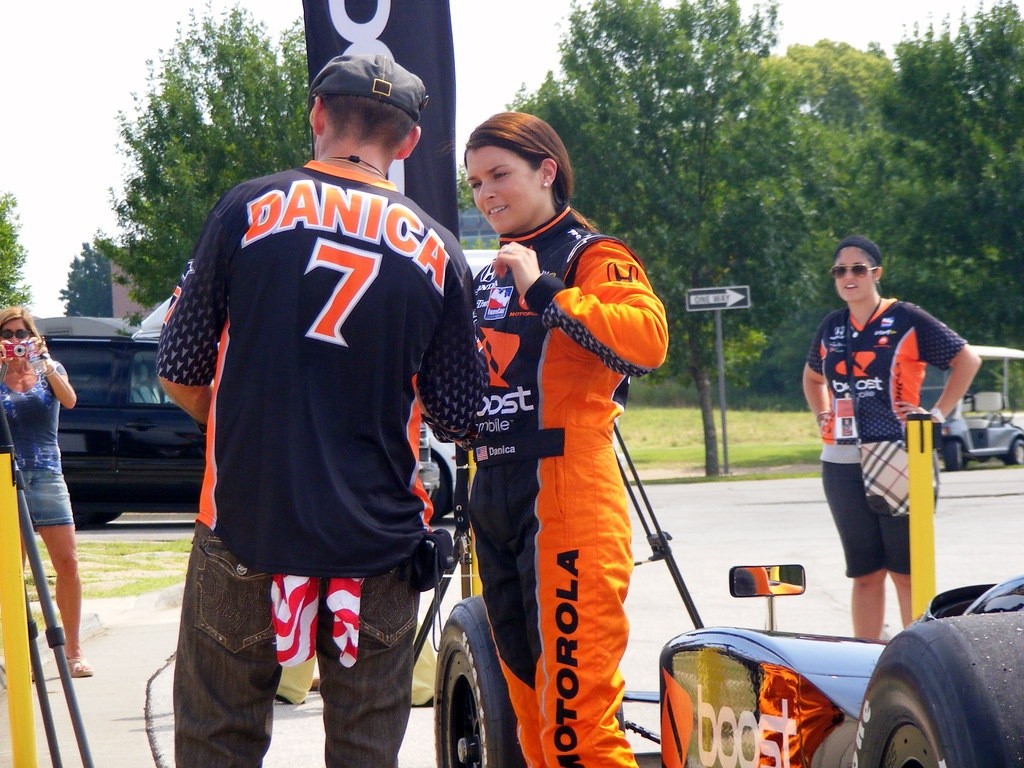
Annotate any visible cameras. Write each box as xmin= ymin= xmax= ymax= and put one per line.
xmin=2 ymin=343 xmax=27 ymax=357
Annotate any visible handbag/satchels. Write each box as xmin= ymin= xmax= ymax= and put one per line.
xmin=857 ymin=433 xmax=908 ymax=516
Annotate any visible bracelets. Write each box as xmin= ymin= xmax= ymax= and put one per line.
xmin=818 ymin=410 xmax=832 ymax=417
xmin=44 ymin=367 xmax=56 ymax=376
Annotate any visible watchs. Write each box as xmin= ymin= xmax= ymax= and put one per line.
xmin=931 ymin=407 xmax=946 ymax=424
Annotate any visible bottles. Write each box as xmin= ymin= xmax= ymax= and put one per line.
xmin=26 ymin=342 xmax=48 ymax=375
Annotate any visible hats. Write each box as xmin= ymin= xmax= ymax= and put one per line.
xmin=307 ymin=53 xmax=429 ymax=123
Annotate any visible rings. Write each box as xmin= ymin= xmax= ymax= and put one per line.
xmin=0 ymin=350 xmax=4 ymax=357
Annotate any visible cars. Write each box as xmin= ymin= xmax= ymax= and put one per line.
xmin=31 ymin=335 xmax=212 ymax=529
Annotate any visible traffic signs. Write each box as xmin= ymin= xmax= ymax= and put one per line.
xmin=686 ymin=285 xmax=752 ymax=312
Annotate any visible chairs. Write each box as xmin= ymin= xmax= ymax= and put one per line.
xmin=966 ymin=391 xmax=1003 ymax=431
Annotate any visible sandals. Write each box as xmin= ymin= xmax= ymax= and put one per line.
xmin=67 ymin=655 xmax=93 ymax=677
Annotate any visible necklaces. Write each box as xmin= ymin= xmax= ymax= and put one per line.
xmin=319 ymin=155 xmax=386 ymax=180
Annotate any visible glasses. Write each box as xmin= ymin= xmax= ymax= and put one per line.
xmin=0 ymin=329 xmax=31 ymax=339
xmin=830 ymin=263 xmax=877 ymax=279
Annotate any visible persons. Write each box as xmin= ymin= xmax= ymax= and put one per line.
xmin=155 ymin=51 xmax=491 ymax=767
xmin=843 ymin=418 xmax=852 ymax=436
xmin=465 ymin=112 xmax=669 ymax=768
xmin=0 ymin=306 xmax=94 ymax=683
xmin=803 ymin=236 xmax=982 ymax=639
